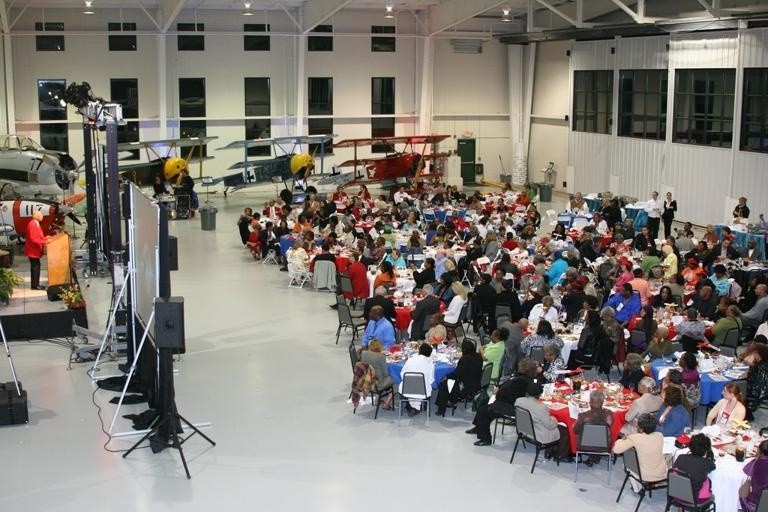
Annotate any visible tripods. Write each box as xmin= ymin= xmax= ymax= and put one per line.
xmin=122 ymin=351 xmax=216 ymax=479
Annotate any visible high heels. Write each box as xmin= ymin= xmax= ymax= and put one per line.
xmin=446 ymin=404 xmax=457 ymax=410
xmin=435 ymin=408 xmax=445 ymax=417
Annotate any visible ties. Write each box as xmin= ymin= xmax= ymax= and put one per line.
xmin=373 ymin=322 xmax=378 ymax=332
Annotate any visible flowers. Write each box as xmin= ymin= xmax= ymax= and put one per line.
xmin=57 ymin=283 xmax=85 ymax=307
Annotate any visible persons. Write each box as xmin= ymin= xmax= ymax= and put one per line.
xmin=24 ymin=210 xmax=53 ymax=290
xmin=173 ymin=169 xmax=195 ymax=198
xmin=154 ymin=176 xmax=170 ymax=198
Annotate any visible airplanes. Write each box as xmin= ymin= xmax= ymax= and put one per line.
xmin=0 ymin=194 xmax=86 ymax=243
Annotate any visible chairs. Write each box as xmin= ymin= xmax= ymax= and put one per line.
xmin=242 ymin=192 xmax=768 ymax=512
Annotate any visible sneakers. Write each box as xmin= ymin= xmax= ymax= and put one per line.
xmin=404 ymin=405 xmax=417 ymax=417
xmin=330 ymin=303 xmax=338 ymax=310
xmin=382 ymin=406 xmax=395 ymax=411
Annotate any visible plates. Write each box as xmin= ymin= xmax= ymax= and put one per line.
xmin=540 ymin=379 xmax=633 ymax=412
xmin=700 ymin=423 xmax=764 ymax=462
xmin=659 ymin=351 xmax=749 ymax=382
xmin=387 ymin=341 xmax=459 ymax=368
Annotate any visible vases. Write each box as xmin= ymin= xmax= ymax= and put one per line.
xmin=68 ymin=302 xmax=81 ymax=310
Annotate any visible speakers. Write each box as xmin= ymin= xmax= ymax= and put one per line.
xmin=168 ymin=236 xmax=178 ymax=270
xmin=155 ymin=296 xmax=185 ymax=348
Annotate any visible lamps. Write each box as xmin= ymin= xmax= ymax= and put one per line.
xmin=498 ymin=1 xmax=514 ymax=23
xmin=382 ymin=2 xmax=396 ymax=19
xmin=240 ymin=1 xmax=256 ymax=16
xmin=81 ymin=1 xmax=97 ymax=15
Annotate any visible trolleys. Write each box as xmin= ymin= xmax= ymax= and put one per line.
xmin=159 ymin=187 xmax=193 ymax=220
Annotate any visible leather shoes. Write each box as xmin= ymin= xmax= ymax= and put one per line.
xmin=465 ymin=428 xmax=477 ymax=434
xmin=474 ymin=439 xmax=492 ymax=446
xmin=553 ymin=454 xmax=575 ymax=463
xmin=583 ymin=458 xmax=600 ymax=467
xmin=631 ymin=486 xmax=645 ymax=496
xmin=544 ymin=453 xmax=554 ymax=460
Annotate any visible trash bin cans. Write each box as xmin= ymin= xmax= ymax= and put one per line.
xmin=538 ymin=182 xmax=554 ymax=202
xmin=198 ymin=206 xmax=217 ymax=230
